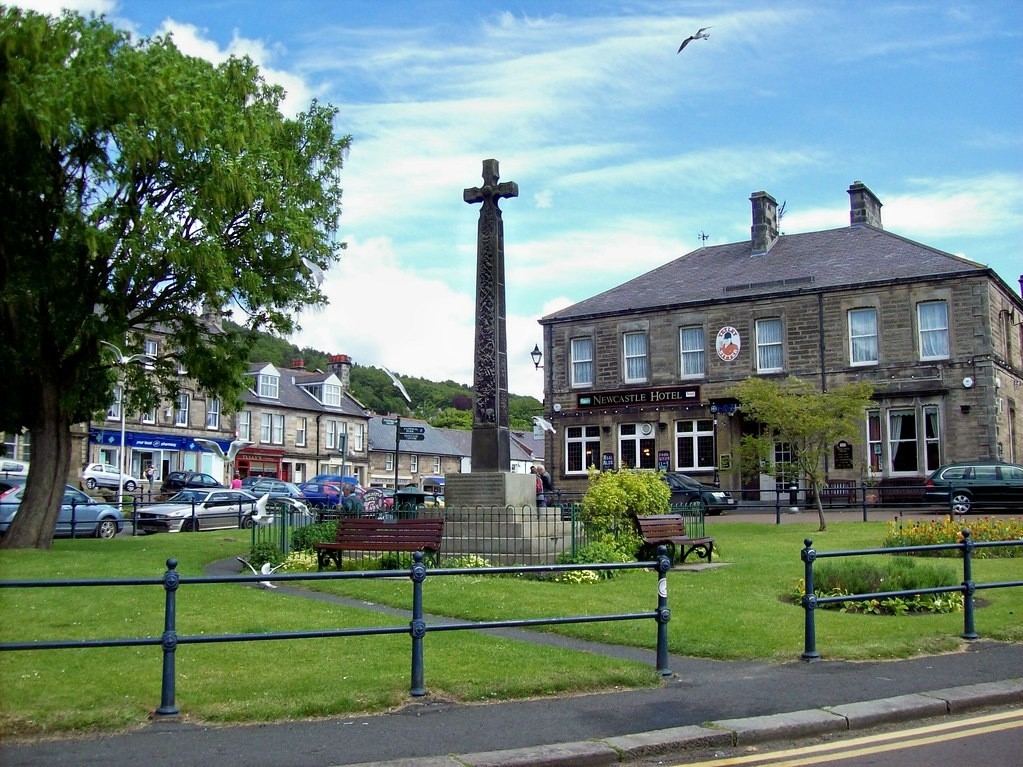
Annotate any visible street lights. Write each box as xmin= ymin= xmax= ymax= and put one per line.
xmin=102 ymin=341 xmax=158 ymax=509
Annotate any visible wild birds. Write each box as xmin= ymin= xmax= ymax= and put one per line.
xmin=241 ymin=493 xmax=282 ymax=532
xmin=378 ymin=363 xmax=412 ymax=403
xmin=276 ymin=496 xmax=313 ymax=516
xmin=529 ymin=416 xmax=557 ymax=433
xmin=235 ymin=556 xmax=284 ymax=590
xmin=677 ymin=25 xmax=712 ymax=53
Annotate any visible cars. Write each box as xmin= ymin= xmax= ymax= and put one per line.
xmin=160 ymin=471 xmax=225 ymax=497
xmin=323 ymin=482 xmax=366 ymax=499
xmin=241 ymin=476 xmax=266 ymax=489
xmin=924 ymin=460 xmax=1023 ymax=515
xmin=241 ymin=480 xmax=304 ymax=500
xmin=0 ymin=475 xmax=124 ymax=539
xmin=0 ymin=458 xmax=30 ymax=476
xmin=360 ymin=487 xmax=394 ymax=513
xmin=82 ymin=463 xmax=138 ymax=492
xmin=660 ymin=472 xmax=738 ymax=517
xmin=131 ymin=488 xmax=258 ymax=534
xmin=299 ymin=483 xmax=347 ymax=509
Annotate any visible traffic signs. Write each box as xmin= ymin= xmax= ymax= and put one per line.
xmin=382 ymin=418 xmax=425 ymax=441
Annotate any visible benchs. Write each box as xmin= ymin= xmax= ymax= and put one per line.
xmin=804 ymin=478 xmax=857 ymax=507
xmin=878 ymin=476 xmax=934 ymax=504
xmin=631 ymin=514 xmax=714 ymax=564
xmin=311 ymin=518 xmax=445 ymax=568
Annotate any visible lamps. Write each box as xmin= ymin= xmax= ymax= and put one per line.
xmin=530 ymin=344 xmax=544 ymax=369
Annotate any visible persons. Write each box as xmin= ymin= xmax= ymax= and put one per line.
xmin=230 ymin=474 xmax=242 ymax=489
xmin=339 ymin=483 xmax=364 ymax=519
xmin=145 ymin=464 xmax=158 ymax=493
xmin=530 ymin=466 xmax=553 ymax=507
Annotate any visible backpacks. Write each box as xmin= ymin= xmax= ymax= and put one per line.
xmin=536 ymin=478 xmax=542 ymax=492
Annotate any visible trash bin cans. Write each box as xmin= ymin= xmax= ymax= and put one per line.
xmin=392 ymin=483 xmax=430 ymax=520
